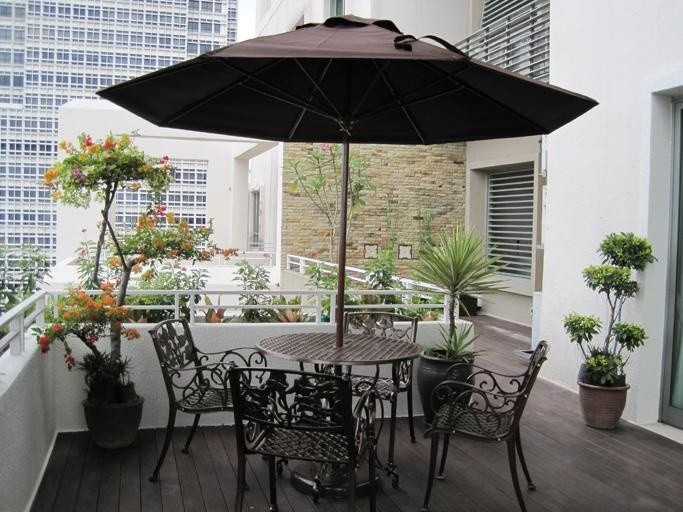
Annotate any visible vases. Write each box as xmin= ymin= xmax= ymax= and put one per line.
xmin=82 ymin=396 xmax=146 ymax=450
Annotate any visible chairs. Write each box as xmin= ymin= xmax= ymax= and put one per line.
xmin=229 ymin=365 xmax=384 ymax=512
xmin=148 ymin=317 xmax=267 ymax=490
xmin=315 ymin=312 xmax=419 ymax=466
xmin=423 ymin=340 xmax=549 ymax=512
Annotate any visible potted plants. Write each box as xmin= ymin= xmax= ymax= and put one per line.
xmin=563 ymin=232 xmax=659 ymax=431
xmin=409 ymin=226 xmax=509 ymax=426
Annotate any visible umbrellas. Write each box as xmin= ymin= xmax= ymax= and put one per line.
xmin=95 ymin=14 xmax=600 ymax=473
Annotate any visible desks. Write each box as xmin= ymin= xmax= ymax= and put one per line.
xmin=257 ymin=333 xmax=426 ymax=500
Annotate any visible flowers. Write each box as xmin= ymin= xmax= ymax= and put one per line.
xmin=30 ymin=133 xmax=241 ymax=403
